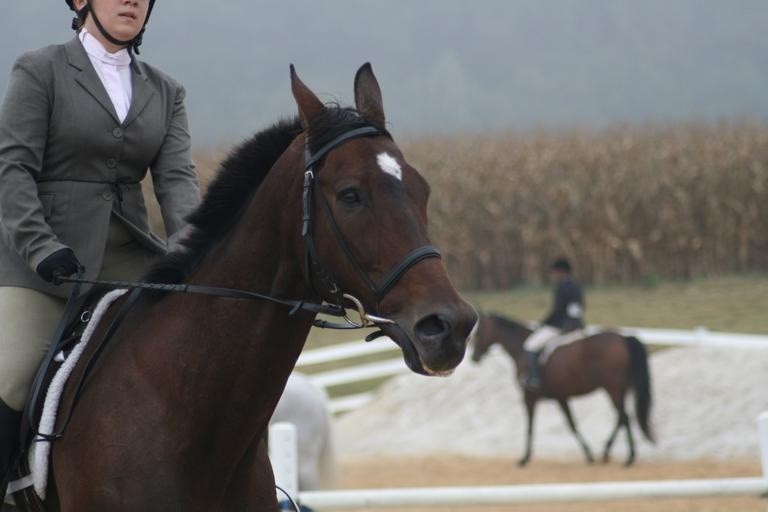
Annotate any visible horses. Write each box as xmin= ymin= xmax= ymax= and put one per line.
xmin=472 ymin=313 xmax=656 ymax=466
xmin=1 ymin=60 xmax=478 ymax=512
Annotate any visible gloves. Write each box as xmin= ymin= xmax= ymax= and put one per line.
xmin=37 ymin=246 xmax=83 ymax=286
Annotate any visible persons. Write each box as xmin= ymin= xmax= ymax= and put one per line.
xmin=0 ymin=0 xmax=206 ymax=511
xmin=517 ymin=255 xmax=587 ymax=393
